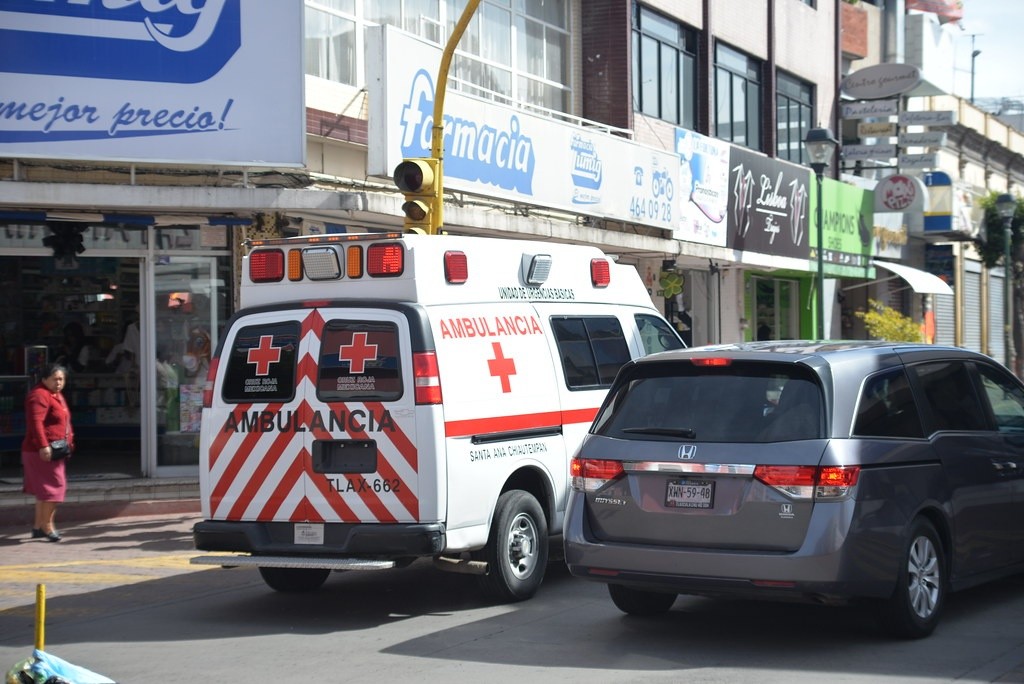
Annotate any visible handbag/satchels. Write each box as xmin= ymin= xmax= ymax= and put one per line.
xmin=50 ymin=439 xmax=72 ymax=461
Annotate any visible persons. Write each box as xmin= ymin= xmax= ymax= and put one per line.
xmin=105 ymin=317 xmax=140 ymax=373
xmin=22 ymin=363 xmax=75 ymax=542
xmin=49 ymin=319 xmax=89 ymax=371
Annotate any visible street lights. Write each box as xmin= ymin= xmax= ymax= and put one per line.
xmin=995 ymin=193 xmax=1017 ymax=374
xmin=971 ymin=50 xmax=984 ymax=108
xmin=802 ymin=120 xmax=841 ymax=341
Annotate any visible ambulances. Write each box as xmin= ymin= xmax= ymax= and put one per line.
xmin=188 ymin=234 xmax=689 ymax=604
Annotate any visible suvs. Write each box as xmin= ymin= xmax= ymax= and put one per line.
xmin=562 ymin=340 xmax=1023 ymax=642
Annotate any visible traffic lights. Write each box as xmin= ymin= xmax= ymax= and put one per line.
xmin=393 ymin=154 xmax=442 ymax=235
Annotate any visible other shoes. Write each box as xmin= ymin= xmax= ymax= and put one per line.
xmin=32 ymin=528 xmax=46 ymax=538
xmin=39 ymin=526 xmax=61 ymax=542
